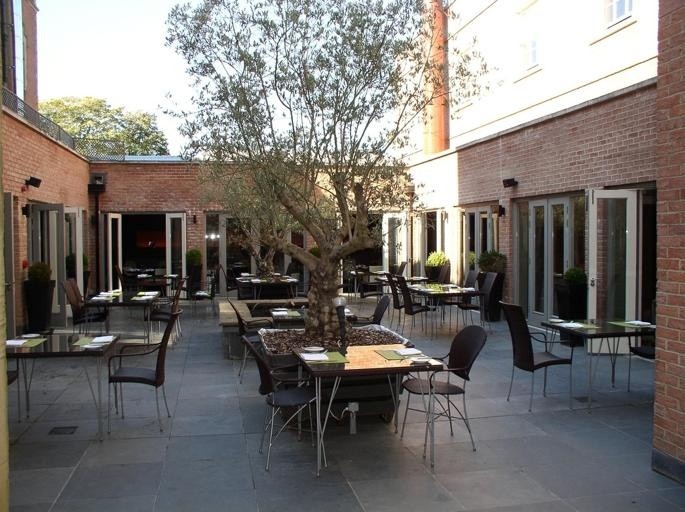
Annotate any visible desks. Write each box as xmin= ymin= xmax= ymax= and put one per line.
xmin=374 ymin=273 xmax=429 ymax=308
xmin=292 ymin=344 xmax=444 ymax=478
xmin=400 ymin=283 xmax=485 ymax=339
xmin=7 ymin=332 xmax=120 ymax=442
xmin=237 ymin=271 xmax=298 ymax=299
xmin=540 ymin=319 xmax=655 ymax=413
xmin=84 ymin=289 xmax=161 ymax=355
xmin=135 ymin=273 xmax=177 ymax=296
xmin=268 ymin=305 xmax=356 ymax=330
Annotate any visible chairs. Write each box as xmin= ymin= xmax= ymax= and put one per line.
xmin=242 ymin=335 xmax=328 ymax=472
xmin=150 ymin=287 xmax=181 ymax=344
xmin=172 ymin=265 xmax=191 ymax=302
xmin=458 ymin=272 xmax=498 ymax=328
xmin=395 ymin=273 xmax=437 ymax=336
xmin=498 ymin=300 xmax=575 ymax=412
xmin=68 ymin=278 xmax=105 ymax=332
xmin=384 ymin=272 xmax=423 ymax=332
xmin=352 ymin=296 xmax=390 ymax=328
xmin=107 ymin=308 xmax=183 ymax=434
xmin=60 ymin=280 xmax=106 ymax=334
xmin=456 ymin=285 xmax=481 ymax=329
xmin=628 ymin=299 xmax=656 ymax=392
xmin=7 ymin=368 xmax=21 ymax=425
xmin=115 ymin=265 xmax=143 ymax=307
xmin=442 ymin=271 xmax=480 ymax=325
xmin=221 ymin=263 xmax=238 ymax=298
xmin=382 ymin=262 xmax=407 ymax=287
xmin=400 ymin=325 xmax=487 ymax=458
xmin=426 ymin=262 xmax=448 ymax=312
xmin=356 ymin=269 xmax=385 ymax=308
xmin=153 ymin=285 xmax=183 ymax=339
xmin=227 ymin=298 xmax=277 ymax=384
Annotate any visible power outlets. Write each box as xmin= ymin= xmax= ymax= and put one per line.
xmin=348 ymin=403 xmax=359 ymax=412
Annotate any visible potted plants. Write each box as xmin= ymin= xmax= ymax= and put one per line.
xmin=22 ymin=261 xmax=55 ymax=330
xmin=554 ymin=267 xmax=588 ymax=347
xmin=477 ymin=250 xmax=507 ymax=323
xmin=422 ymin=250 xmax=450 ymax=306
xmin=184 ymin=249 xmax=202 ymax=298
xmin=66 ymin=253 xmax=91 ymax=304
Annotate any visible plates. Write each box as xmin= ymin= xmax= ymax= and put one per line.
xmin=83 ymin=344 xmax=104 ymax=350
xmin=548 ymin=317 xmax=564 ymax=323
xmin=304 ymin=346 xmax=325 ymax=353
xmin=22 ymin=333 xmax=40 ymax=338
xmin=411 ymin=357 xmax=430 ymax=364
xmin=638 ymin=322 xmax=651 ymax=326
